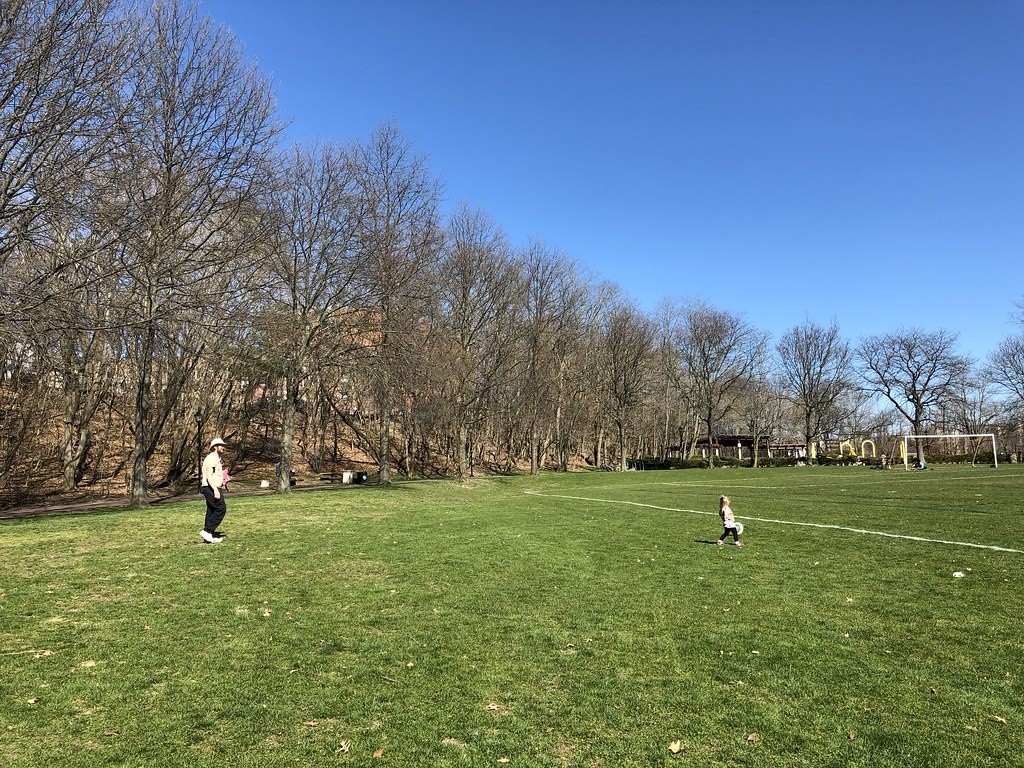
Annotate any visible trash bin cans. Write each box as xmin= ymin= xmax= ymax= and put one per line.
xmin=342 ymin=470 xmax=353 ymax=484
xmin=357 ymin=471 xmax=367 ymax=484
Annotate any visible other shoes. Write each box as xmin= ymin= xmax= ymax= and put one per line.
xmin=736 ymin=542 xmax=745 ymax=547
xmin=717 ymin=539 xmax=725 ymax=545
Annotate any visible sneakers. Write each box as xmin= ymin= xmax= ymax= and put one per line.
xmin=199 ymin=530 xmax=223 ymax=543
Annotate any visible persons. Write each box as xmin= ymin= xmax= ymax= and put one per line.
xmin=717 ymin=495 xmax=744 ymax=546
xmin=198 ymin=437 xmax=228 ymax=544
xmin=911 ymin=459 xmax=927 ymax=470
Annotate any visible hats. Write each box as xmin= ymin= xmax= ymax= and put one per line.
xmin=209 ymin=438 xmax=227 ymax=452
xmin=734 ymin=522 xmax=744 ymax=535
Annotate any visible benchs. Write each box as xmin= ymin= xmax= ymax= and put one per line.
xmin=312 ymin=472 xmax=342 ymax=484
